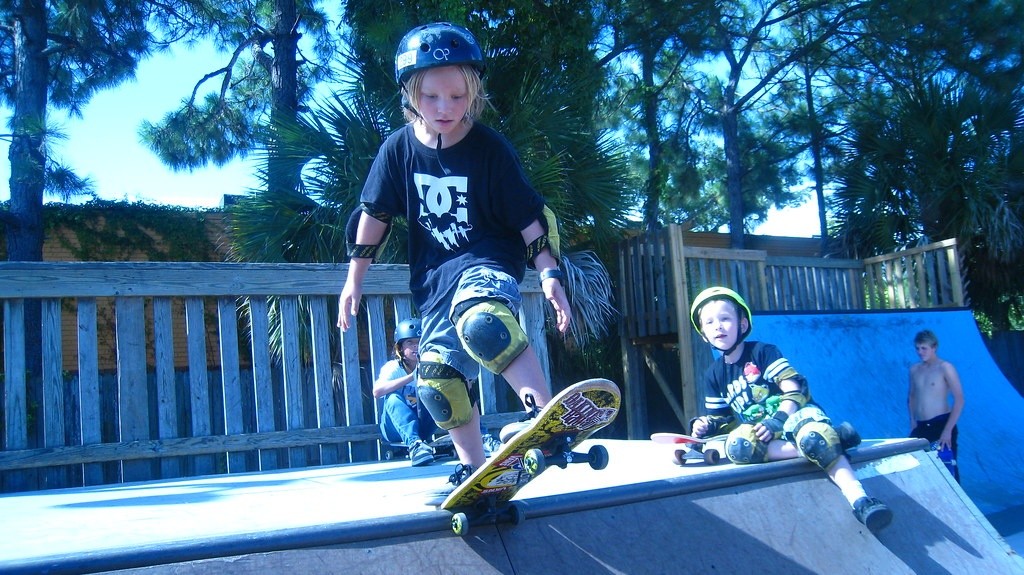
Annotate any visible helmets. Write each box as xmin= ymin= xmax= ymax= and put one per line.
xmin=691 ymin=287 xmax=751 ymax=340
xmin=394 ymin=23 xmax=486 ymax=86
xmin=393 ymin=318 xmax=422 ymax=343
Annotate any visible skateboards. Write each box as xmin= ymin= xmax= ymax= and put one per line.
xmin=929 ymin=441 xmax=957 ymax=478
xmin=439 ymin=377 xmax=622 ymax=535
xmin=650 ymin=432 xmax=729 ymax=464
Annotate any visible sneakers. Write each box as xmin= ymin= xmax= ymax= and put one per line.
xmin=479 ymin=432 xmax=502 ymax=456
xmin=406 ymin=441 xmax=434 ymax=466
xmin=424 ymin=463 xmax=479 ymax=505
xmin=851 ymin=497 xmax=892 ymax=529
xmin=833 ymin=423 xmax=861 ymax=450
xmin=499 ymin=393 xmax=543 ymax=444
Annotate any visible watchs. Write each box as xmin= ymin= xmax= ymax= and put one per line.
xmin=538 ymin=271 xmax=565 ymax=288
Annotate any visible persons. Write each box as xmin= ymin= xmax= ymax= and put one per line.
xmin=374 ymin=319 xmax=503 ymax=466
xmin=691 ymin=286 xmax=893 ymax=529
xmin=336 ymin=22 xmax=570 ymax=506
xmin=908 ymin=329 xmax=965 ymax=483
